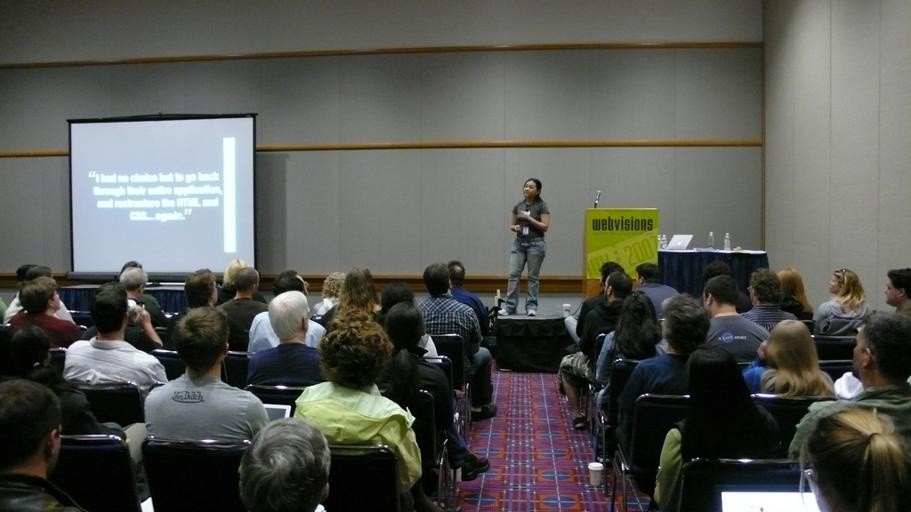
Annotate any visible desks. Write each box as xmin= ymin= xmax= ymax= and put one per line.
xmin=658 ymin=247 xmax=771 ymax=299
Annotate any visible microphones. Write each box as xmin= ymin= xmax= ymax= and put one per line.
xmin=595 ymin=191 xmax=600 ymax=204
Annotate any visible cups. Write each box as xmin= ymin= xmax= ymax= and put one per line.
xmin=562 ymin=303 xmax=572 ymax=319
xmin=587 ymin=462 xmax=603 ymax=488
xmin=126 ymin=298 xmax=143 ymax=324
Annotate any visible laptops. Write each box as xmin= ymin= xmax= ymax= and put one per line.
xmin=666 ymin=235 xmax=694 ymax=250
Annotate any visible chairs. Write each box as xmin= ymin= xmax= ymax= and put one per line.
xmin=581 ymin=314 xmax=907 ymax=512
xmin=1 ymin=281 xmax=497 ymax=511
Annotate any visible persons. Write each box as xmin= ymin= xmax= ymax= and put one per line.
xmin=497 ymin=178 xmax=551 ymax=316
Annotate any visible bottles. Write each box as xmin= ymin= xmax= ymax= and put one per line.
xmin=707 ymin=231 xmax=715 ymax=249
xmin=661 ymin=234 xmax=668 ymax=250
xmin=657 ymin=234 xmax=661 ymax=250
xmin=723 ymin=232 xmax=731 ymax=250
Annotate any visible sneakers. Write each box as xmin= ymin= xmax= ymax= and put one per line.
xmin=500 ymin=306 xmax=516 ymax=316
xmin=461 ymin=457 xmax=492 ymax=482
xmin=574 ymin=415 xmax=586 ymax=429
xmin=527 ymin=307 xmax=537 ymax=317
xmin=596 ymin=446 xmax=612 ymax=464
xmin=471 ymin=404 xmax=497 ymax=421
